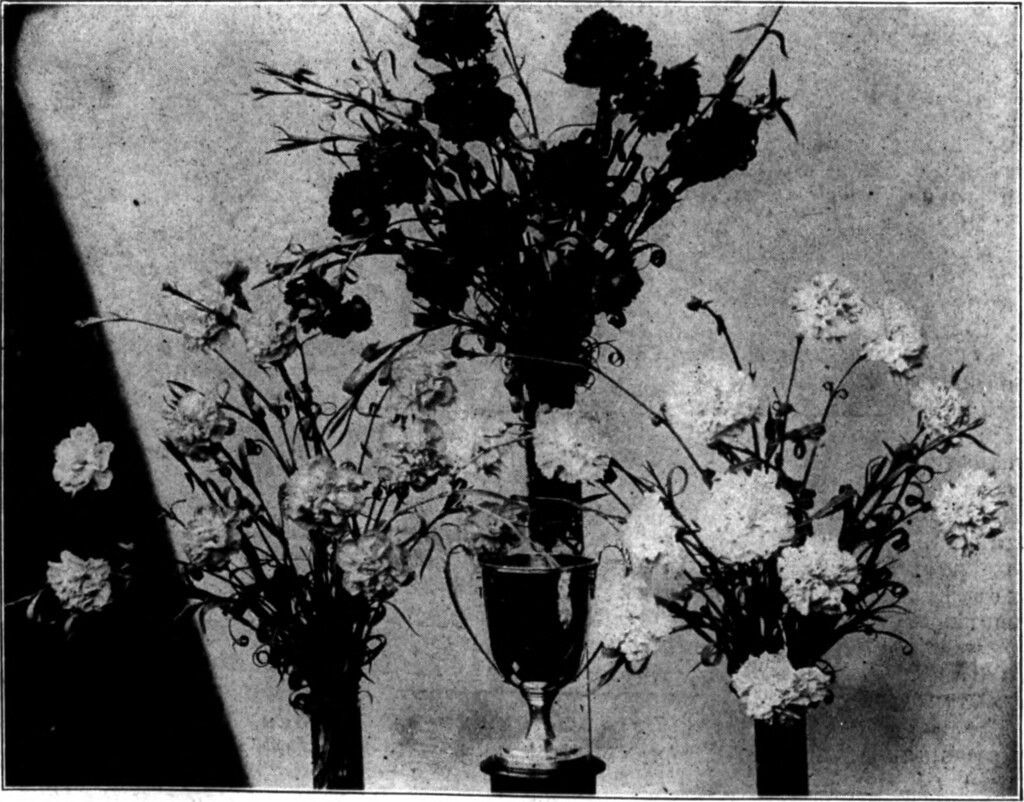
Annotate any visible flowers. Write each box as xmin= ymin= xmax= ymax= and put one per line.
xmin=8 ymin=5 xmax=1013 ymax=728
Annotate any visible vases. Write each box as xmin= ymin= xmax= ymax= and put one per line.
xmin=310 ymin=700 xmax=363 ymax=790
xmin=755 ymin=714 xmax=808 ymax=795
xmin=480 ymin=550 xmax=599 ymax=772
